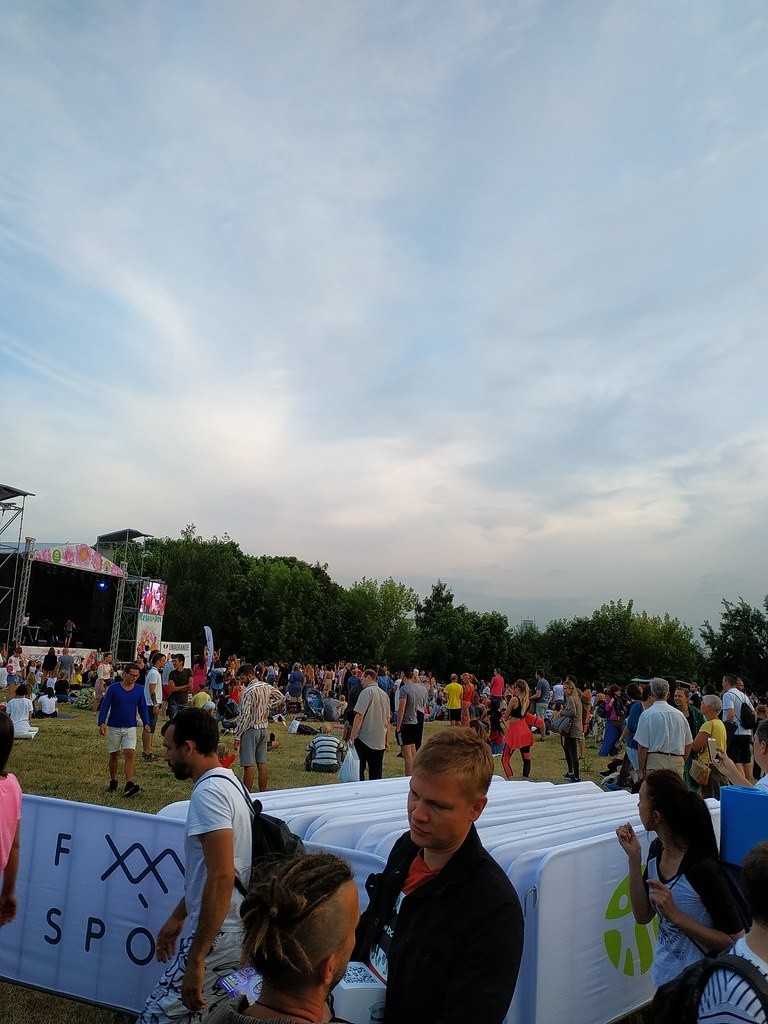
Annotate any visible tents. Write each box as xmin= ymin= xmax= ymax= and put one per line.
xmin=0 ymin=542 xmax=127 ymax=669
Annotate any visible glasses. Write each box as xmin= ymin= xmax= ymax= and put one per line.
xmin=129 ymin=673 xmax=140 ymax=678
xmin=752 ymin=736 xmax=768 ymax=744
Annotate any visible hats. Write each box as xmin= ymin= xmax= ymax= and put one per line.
xmin=258 ymin=662 xmax=264 ymax=667
xmin=352 ymin=663 xmax=358 ymax=667
xmin=328 ymin=690 xmax=334 ymax=695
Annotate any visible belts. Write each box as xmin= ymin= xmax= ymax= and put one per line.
xmin=647 ymin=751 xmax=683 ymax=756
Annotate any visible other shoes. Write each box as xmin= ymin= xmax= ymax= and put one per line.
xmin=397 ymin=753 xmax=402 ymax=757
xmin=143 ymin=752 xmax=159 ymax=762
xmin=270 ymin=733 xmax=275 ymax=741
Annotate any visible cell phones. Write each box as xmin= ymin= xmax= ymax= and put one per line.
xmin=707 ymin=737 xmax=720 ymax=763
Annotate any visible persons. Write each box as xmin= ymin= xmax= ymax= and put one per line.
xmin=167 ymin=654 xmax=195 ymax=720
xmin=304 ymin=721 xmax=348 ymax=773
xmin=443 ymin=673 xmax=464 ymax=726
xmin=22 ymin=609 xmax=32 ymax=644
xmin=616 ymin=768 xmax=746 ymax=990
xmin=0 ymin=644 xmax=768 ymax=799
xmin=349 ymin=669 xmax=391 ymax=781
xmin=395 ymin=662 xmax=419 ymax=776
xmin=561 ymin=680 xmax=583 ymax=783
xmin=0 ymin=708 xmax=22 ymax=930
xmin=134 ymin=706 xmax=255 ymax=1024
xmin=142 ymin=653 xmax=166 ymax=761
xmin=498 ymin=679 xmax=546 ymax=780
xmin=349 ymin=727 xmax=524 ymax=1024
xmin=698 ymin=843 xmax=768 ymax=1024
xmin=197 ymin=850 xmax=361 ymax=1024
xmin=142 ymin=586 xmax=167 ymax=615
xmin=64 ymin=616 xmax=76 ymax=647
xmin=234 ymin=664 xmax=287 ymax=792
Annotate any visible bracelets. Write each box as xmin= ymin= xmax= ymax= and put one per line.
xmin=153 ymin=704 xmax=160 ymax=707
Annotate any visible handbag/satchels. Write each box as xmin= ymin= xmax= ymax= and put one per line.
xmin=689 ymin=759 xmax=712 ymax=785
xmin=338 ymin=739 xmax=360 ymax=782
xmin=288 ymin=716 xmax=302 ymax=733
xmin=550 ymin=716 xmax=572 ymax=735
xmin=597 ymin=702 xmax=611 ymax=718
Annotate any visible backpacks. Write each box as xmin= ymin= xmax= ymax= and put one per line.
xmin=194 ymin=773 xmax=306 ymax=897
xmin=298 ymin=724 xmax=317 ymax=735
xmin=213 ymin=671 xmax=223 ymax=684
xmin=223 ymin=699 xmax=240 ymax=720
xmin=729 ymin=692 xmax=755 ymax=730
xmin=644 ymin=937 xmax=768 ymax=1024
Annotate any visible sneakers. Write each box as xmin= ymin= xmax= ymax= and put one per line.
xmin=562 ymin=772 xmax=573 ymax=780
xmin=569 ymin=777 xmax=580 ymax=783
xmin=124 ymin=781 xmax=140 ymax=797
xmin=598 ymin=770 xmax=610 ymax=777
xmin=107 ymin=780 xmax=118 ymax=793
xmin=604 ymin=783 xmax=622 ymax=791
